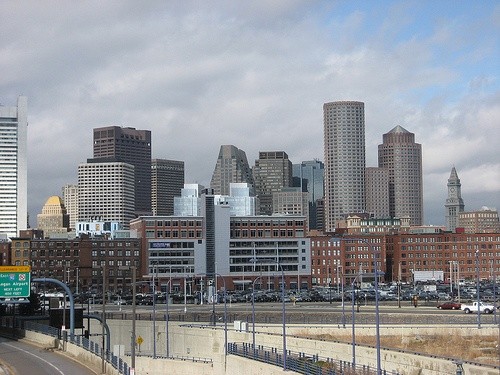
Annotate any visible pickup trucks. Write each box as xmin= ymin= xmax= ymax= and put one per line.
xmin=460 ymin=301 xmax=496 ymax=315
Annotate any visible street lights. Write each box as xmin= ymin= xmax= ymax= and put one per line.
xmin=474 ymin=250 xmax=484 ymax=329
xmin=166 ymin=277 xmax=176 ymax=357
xmin=351 ymin=271 xmax=385 ymax=374
xmin=251 ymin=275 xmax=265 ymax=352
xmin=249 ymin=257 xmax=287 ymax=371
xmin=331 ymin=238 xmax=383 ymax=375
xmin=197 ymin=272 xmax=228 ymax=356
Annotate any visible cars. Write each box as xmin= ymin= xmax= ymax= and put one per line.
xmin=88 ymin=278 xmax=500 ymax=306
xmin=437 ymin=301 xmax=461 ymax=310
